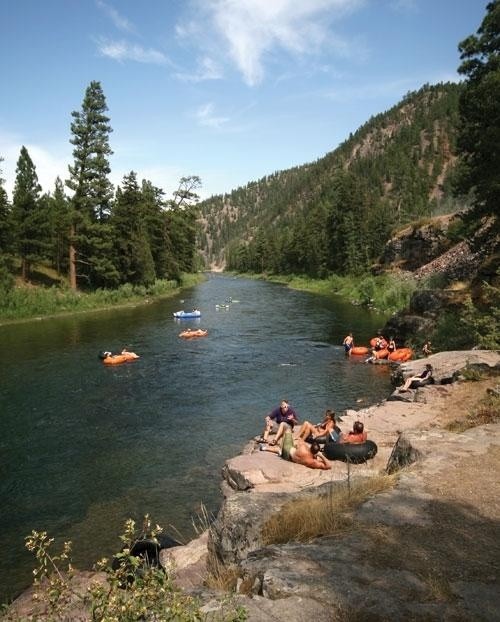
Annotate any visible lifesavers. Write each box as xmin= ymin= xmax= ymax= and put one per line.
xmin=323 ymin=439 xmax=377 ymax=463
xmin=374 ymin=349 xmax=389 ymax=360
xmin=351 ymin=346 xmax=367 ymax=355
xmin=299 ymin=425 xmax=341 ymax=444
xmin=370 ymin=337 xmax=389 ymax=347
xmin=181 ymin=330 xmax=206 ymax=336
xmin=105 ymin=355 xmax=135 ymax=364
xmin=409 ymin=376 xmax=434 ymax=388
xmin=388 ymin=347 xmax=413 ymax=363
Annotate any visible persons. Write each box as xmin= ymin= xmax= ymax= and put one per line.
xmin=399 ymin=364 xmax=433 ymax=392
xmin=364 ymin=350 xmax=377 ymax=362
xmin=194 ymin=328 xmax=207 ymax=335
xmin=386 ymin=335 xmax=396 ymax=359
xmin=422 ymin=341 xmax=435 ymax=354
xmin=121 ymin=348 xmax=140 ymax=358
xmin=256 ymin=399 xmax=298 ymax=446
xmin=179 ymin=329 xmax=192 ymax=337
xmin=104 ymin=353 xmax=118 ymax=358
xmin=318 ymin=421 xmax=368 ymax=452
xmin=295 ymin=409 xmax=336 ymax=442
xmin=259 ymin=429 xmax=332 ymax=470
xmin=343 ymin=333 xmax=355 ymax=356
xmin=376 ymin=336 xmax=384 ymax=350
